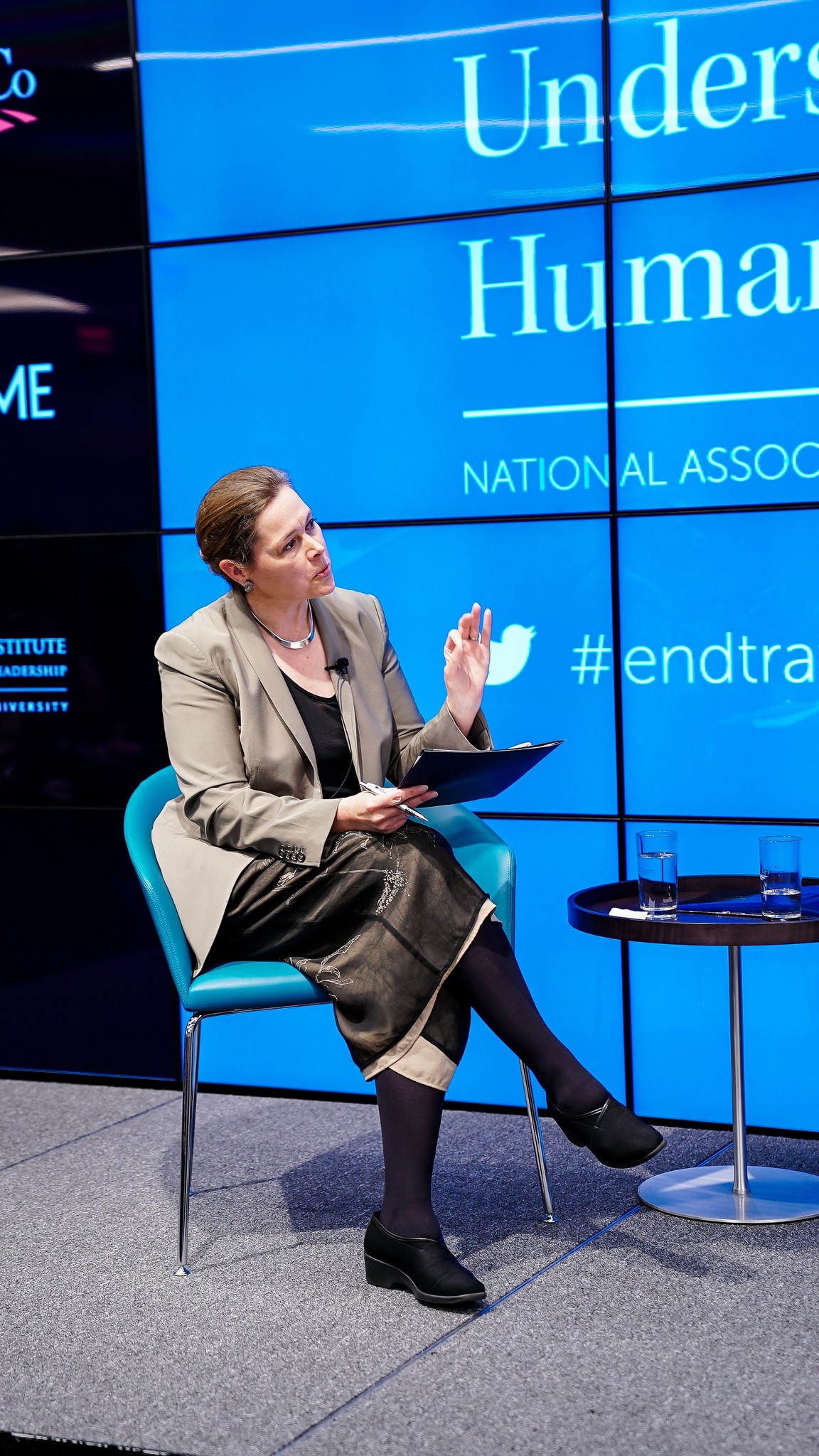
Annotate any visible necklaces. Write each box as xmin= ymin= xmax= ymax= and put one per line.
xmin=249 ymin=599 xmax=315 ymax=649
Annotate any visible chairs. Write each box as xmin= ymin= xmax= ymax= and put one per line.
xmin=116 ymin=763 xmax=564 ymax=1280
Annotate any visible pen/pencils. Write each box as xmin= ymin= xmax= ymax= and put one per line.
xmin=358 ymin=779 xmax=432 ymax=825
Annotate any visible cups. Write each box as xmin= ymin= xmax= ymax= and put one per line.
xmin=759 ymin=836 xmax=803 ymax=923
xmin=636 ymin=830 xmax=679 ymax=918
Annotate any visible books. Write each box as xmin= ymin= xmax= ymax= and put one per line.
xmin=396 ymin=740 xmax=566 ymax=809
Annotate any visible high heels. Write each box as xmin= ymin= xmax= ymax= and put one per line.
xmin=545 ymin=1093 xmax=667 ymax=1169
xmin=362 ymin=1212 xmax=488 ymax=1305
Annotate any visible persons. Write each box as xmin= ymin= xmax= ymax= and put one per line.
xmin=154 ymin=465 xmax=668 ymax=1307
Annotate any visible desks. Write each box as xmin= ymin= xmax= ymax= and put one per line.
xmin=562 ymin=871 xmax=819 ymax=1228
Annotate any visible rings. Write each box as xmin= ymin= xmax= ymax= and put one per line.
xmin=469 ymin=636 xmax=479 ymax=640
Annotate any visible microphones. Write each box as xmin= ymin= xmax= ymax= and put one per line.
xmin=336 ymin=657 xmax=349 ymax=674
